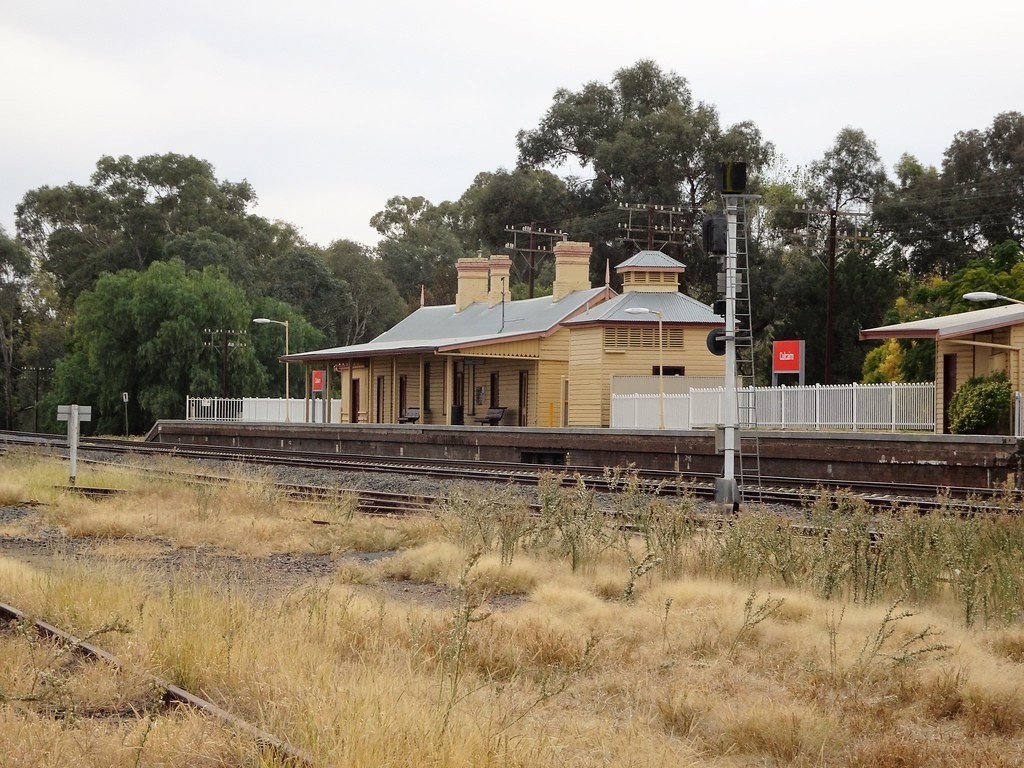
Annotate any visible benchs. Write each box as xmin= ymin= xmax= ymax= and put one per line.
xmin=397 ymin=407 xmax=420 ymax=424
xmin=473 ymin=407 xmax=508 ymax=426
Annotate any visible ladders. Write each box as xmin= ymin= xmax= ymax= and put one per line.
xmin=723 ymin=198 xmax=762 ymax=510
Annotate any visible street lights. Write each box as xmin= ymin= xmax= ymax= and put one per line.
xmin=252 ymin=318 xmax=290 ymax=423
xmin=624 ymin=307 xmax=665 ymax=429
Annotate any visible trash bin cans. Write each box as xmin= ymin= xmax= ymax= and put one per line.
xmin=451 ymin=405 xmax=464 ymax=424
